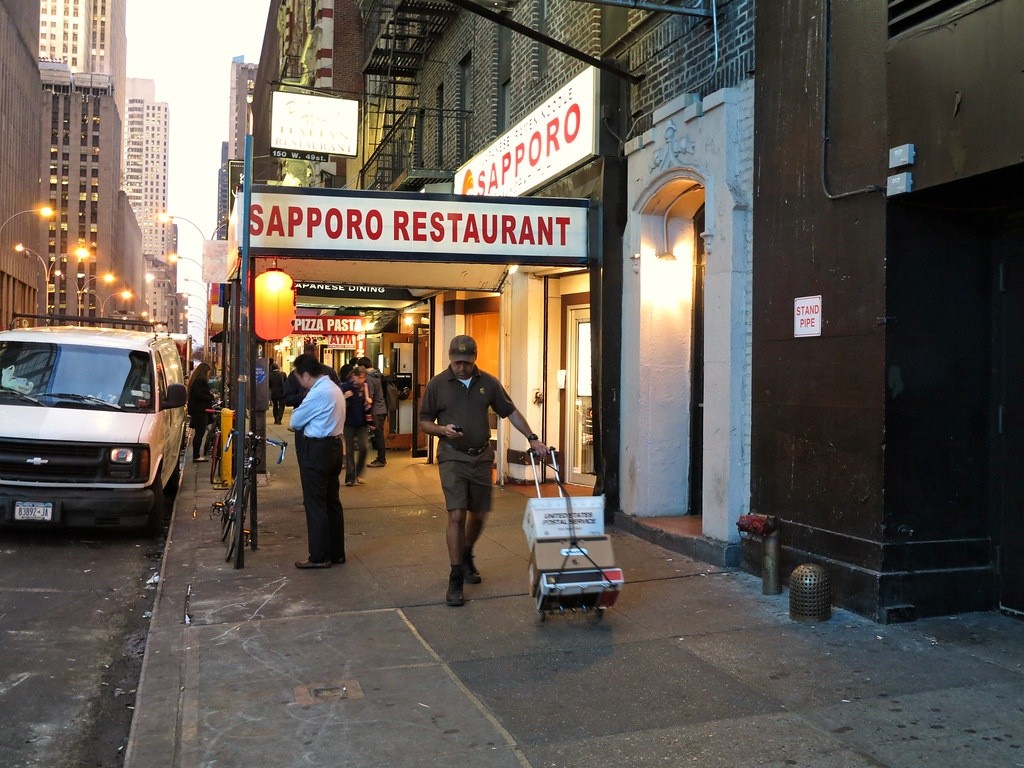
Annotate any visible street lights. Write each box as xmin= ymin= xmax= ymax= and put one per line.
xmin=87 ymin=290 xmax=129 ymax=327
xmin=14 ymin=244 xmax=87 ymax=325
xmin=158 ymin=215 xmax=225 ymax=360
xmin=53 ymin=270 xmax=112 ymax=328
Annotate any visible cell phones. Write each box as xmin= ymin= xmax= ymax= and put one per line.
xmin=453 ymin=426 xmax=463 ymax=433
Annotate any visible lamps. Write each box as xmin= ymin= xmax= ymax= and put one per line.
xmin=658 ymin=252 xmax=677 ymax=262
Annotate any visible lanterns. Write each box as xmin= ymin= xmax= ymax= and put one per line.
xmin=254 ymin=267 xmax=297 ymax=344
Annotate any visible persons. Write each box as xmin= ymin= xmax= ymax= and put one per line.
xmin=420 ymin=335 xmax=548 ymax=607
xmin=289 ymin=354 xmax=345 ymax=568
xmin=187 ymin=362 xmax=218 ymax=462
xmin=269 ymin=344 xmax=389 ymax=486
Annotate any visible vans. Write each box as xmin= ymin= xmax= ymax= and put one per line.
xmin=0 ymin=325 xmax=188 ymax=538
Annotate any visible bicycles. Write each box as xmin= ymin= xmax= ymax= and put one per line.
xmin=204 ymin=409 xmax=235 ymax=482
xmin=209 ymin=428 xmax=288 ymax=562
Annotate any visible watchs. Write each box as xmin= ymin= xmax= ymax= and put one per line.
xmin=528 ymin=434 xmax=538 ymax=442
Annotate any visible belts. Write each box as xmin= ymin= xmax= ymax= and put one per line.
xmin=448 ymin=443 xmax=489 ymax=456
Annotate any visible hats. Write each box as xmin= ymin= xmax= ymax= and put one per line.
xmin=449 ymin=335 xmax=477 ymax=363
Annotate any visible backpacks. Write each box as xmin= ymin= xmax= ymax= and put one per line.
xmin=382 ymin=375 xmax=399 ymax=412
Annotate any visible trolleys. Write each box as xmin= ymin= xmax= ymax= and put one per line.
xmin=525 ymin=446 xmax=612 ymax=624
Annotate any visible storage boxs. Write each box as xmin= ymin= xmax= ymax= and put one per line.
xmin=527 ymin=534 xmax=616 ymax=598
xmin=521 ymin=496 xmax=605 ymax=551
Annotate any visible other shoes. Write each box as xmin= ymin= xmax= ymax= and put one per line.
xmin=462 ymin=555 xmax=481 ymax=584
xmin=345 ymin=476 xmax=367 ymax=486
xmin=295 ymin=554 xmax=345 ymax=569
xmin=193 ymin=457 xmax=209 ymax=462
xmin=367 ymin=459 xmax=386 ymax=467
xmin=445 ymin=572 xmax=464 ymax=606
